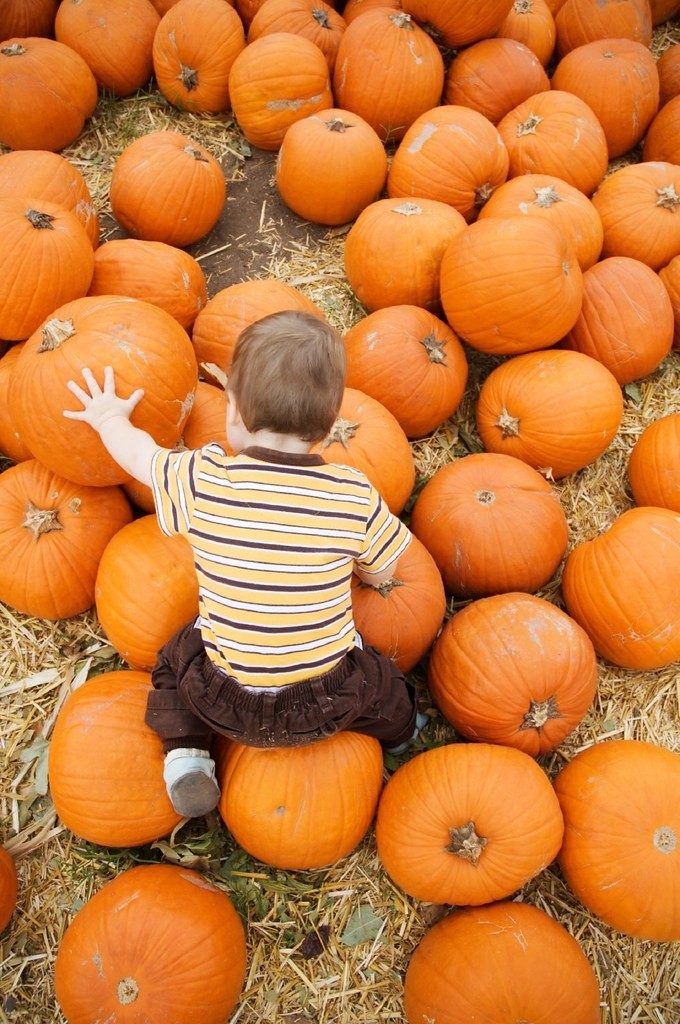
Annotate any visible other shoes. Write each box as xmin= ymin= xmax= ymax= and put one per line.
xmin=387 ymin=710 xmax=430 ymax=756
xmin=163 ymin=746 xmax=224 ymax=814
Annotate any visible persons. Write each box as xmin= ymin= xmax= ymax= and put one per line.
xmin=64 ymin=306 xmax=430 ymax=819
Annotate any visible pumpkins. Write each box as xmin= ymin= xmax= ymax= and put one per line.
xmin=0 ymin=0 xmax=680 ymax=1024
